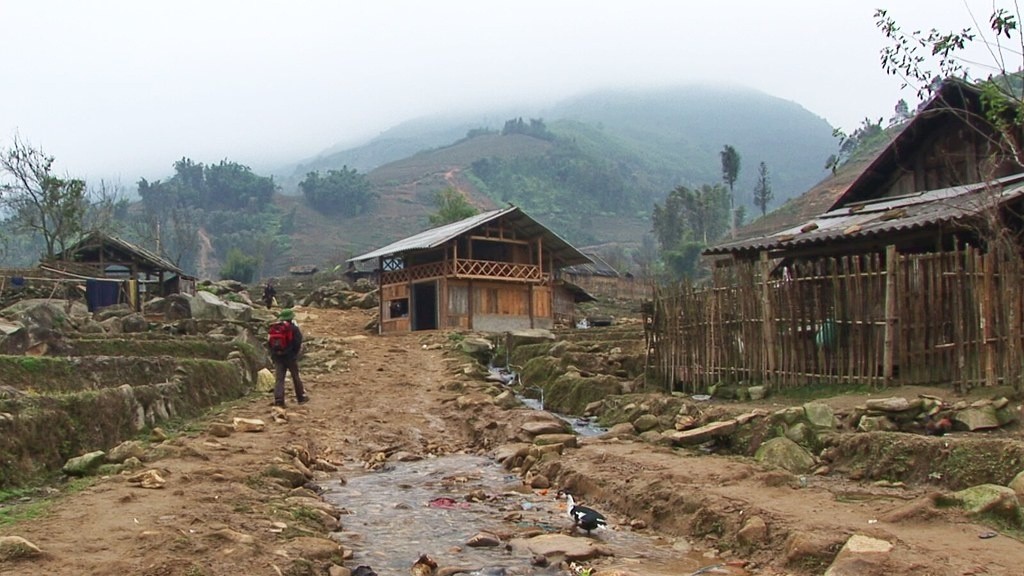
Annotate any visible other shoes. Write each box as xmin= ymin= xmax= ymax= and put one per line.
xmin=298 ymin=396 xmax=309 ymax=405
xmin=275 ymin=402 xmax=286 ymax=408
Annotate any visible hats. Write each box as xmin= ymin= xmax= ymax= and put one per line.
xmin=279 ymin=308 xmax=295 ymax=321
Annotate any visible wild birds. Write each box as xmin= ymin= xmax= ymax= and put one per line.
xmin=555 ymin=490 xmax=607 ymax=535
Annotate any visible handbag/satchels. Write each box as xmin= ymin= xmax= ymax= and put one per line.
xmin=271 ymin=297 xmax=278 ymax=307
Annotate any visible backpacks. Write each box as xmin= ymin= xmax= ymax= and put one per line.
xmin=269 ymin=320 xmax=294 ymax=350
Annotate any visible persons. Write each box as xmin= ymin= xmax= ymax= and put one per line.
xmin=269 ymin=309 xmax=309 ymax=406
xmin=263 ymin=283 xmax=276 ymax=310
